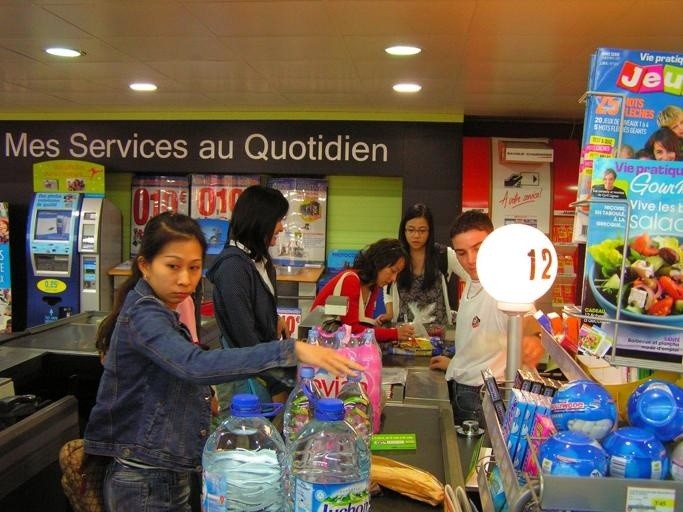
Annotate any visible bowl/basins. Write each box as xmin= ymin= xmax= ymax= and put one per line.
xmin=588 ymin=233 xmax=683 ymax=337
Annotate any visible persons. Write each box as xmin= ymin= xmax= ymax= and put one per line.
xmin=615 ymin=104 xmax=683 ymax=161
xmin=429 ymin=209 xmax=544 ymax=430
xmin=205 ymin=186 xmax=291 ymax=433
xmin=591 ymin=169 xmax=627 ymax=200
xmin=83 ymin=212 xmax=370 ymax=512
xmin=305 ymin=239 xmax=415 ymax=342
xmin=384 ymin=203 xmax=469 ymax=326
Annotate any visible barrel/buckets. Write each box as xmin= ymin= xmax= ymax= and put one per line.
xmin=199 ymin=392 xmax=286 ymax=511
xmin=282 ymin=384 xmax=373 ymax=512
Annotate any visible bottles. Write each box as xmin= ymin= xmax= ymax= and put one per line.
xmin=291 ymin=397 xmax=370 ymax=511
xmin=201 ymin=393 xmax=291 ymax=511
xmin=283 ymin=325 xmax=383 ymax=465
xmin=457 ymin=420 xmax=483 ymax=479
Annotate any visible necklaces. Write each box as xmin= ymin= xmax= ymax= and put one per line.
xmin=465 ymin=279 xmax=484 ymax=300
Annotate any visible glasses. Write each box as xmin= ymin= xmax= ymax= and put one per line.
xmin=405 ymin=227 xmax=429 ymax=235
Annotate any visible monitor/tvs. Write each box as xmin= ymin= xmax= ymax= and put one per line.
xmin=34 ymin=208 xmax=72 ymax=241
